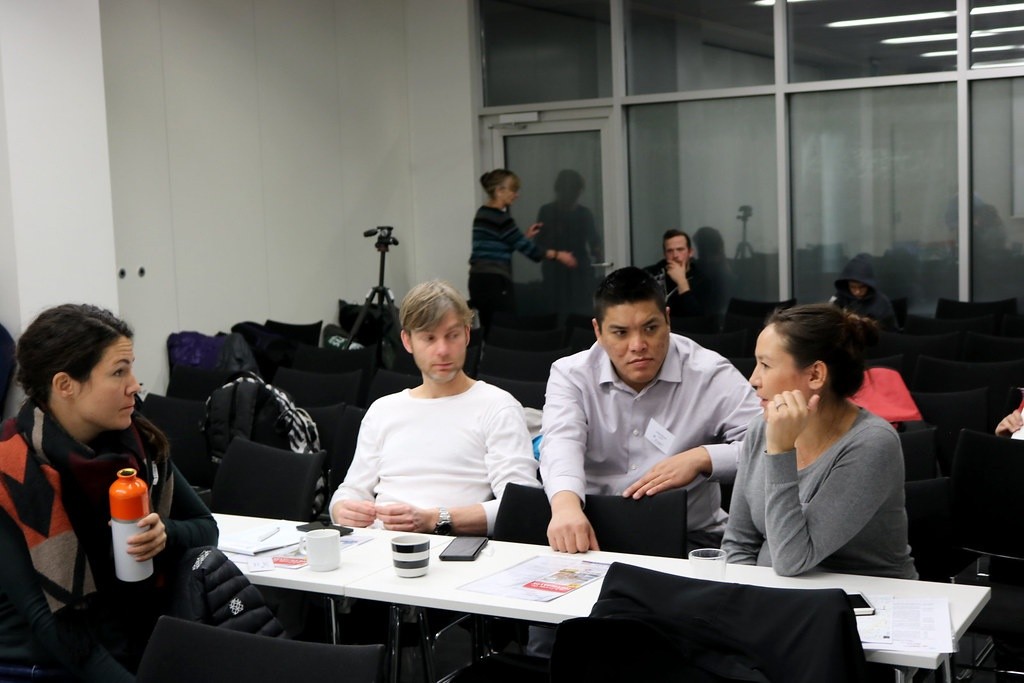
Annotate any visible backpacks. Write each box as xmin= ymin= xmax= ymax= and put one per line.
xmin=199 ymin=374 xmax=321 ymax=490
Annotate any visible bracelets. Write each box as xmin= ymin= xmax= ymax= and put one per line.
xmin=553 ymin=251 xmax=557 ymax=261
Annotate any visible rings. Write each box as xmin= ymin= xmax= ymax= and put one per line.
xmin=776 ymin=402 xmax=788 ymax=411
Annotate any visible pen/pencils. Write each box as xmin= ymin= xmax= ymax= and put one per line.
xmin=258 ymin=528 xmax=279 ymax=542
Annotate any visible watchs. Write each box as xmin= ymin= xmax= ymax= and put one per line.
xmin=435 ymin=508 xmax=452 ymax=534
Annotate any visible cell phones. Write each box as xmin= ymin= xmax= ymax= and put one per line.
xmin=845 ymin=591 xmax=875 ymax=615
xmin=439 ymin=536 xmax=488 ymax=562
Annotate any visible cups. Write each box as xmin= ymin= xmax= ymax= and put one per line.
xmin=299 ymin=528 xmax=341 ymax=572
xmin=689 ymin=549 xmax=726 ymax=583
xmin=390 ymin=535 xmax=431 ymax=578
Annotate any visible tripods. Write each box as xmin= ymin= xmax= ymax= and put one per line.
xmin=343 ymin=238 xmax=404 ymax=365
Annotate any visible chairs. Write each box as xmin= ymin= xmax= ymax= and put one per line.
xmin=0 ymin=297 xmax=1024 ymax=683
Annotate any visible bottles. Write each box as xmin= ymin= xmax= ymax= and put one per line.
xmin=108 ymin=468 xmax=154 ymax=583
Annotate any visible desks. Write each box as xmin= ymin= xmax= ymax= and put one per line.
xmin=212 ymin=514 xmax=992 ymax=683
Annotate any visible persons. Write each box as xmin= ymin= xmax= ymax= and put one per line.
xmin=643 ymin=229 xmax=714 ymax=336
xmin=330 ymin=281 xmax=542 ymax=534
xmin=0 ymin=304 xmax=219 ymax=683
xmin=830 ymin=253 xmax=898 ymax=333
xmin=995 ymin=387 xmax=1024 ymax=440
xmin=538 ymin=266 xmax=764 ymax=554
xmin=463 ymin=170 xmax=578 ymax=377
xmin=721 ymin=304 xmax=919 ymax=579
